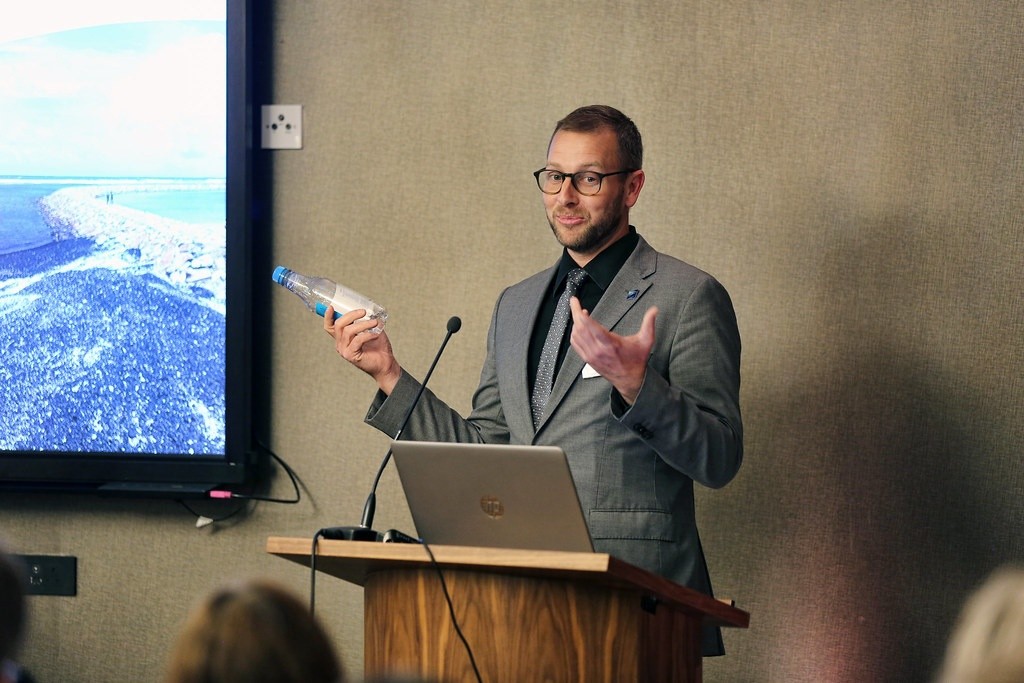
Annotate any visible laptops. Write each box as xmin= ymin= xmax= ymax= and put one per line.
xmin=391 ymin=440 xmax=595 ymax=555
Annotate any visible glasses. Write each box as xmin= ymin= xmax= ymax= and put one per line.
xmin=532 ymin=167 xmax=640 ymax=197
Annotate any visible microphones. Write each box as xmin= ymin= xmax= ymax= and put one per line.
xmin=320 ymin=316 xmax=462 ymax=543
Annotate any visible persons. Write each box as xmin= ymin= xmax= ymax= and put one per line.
xmin=161 ymin=579 xmax=349 ymax=683
xmin=322 ymin=106 xmax=744 ymax=682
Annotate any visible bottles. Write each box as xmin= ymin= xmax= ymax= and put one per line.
xmin=271 ymin=266 xmax=389 ymax=338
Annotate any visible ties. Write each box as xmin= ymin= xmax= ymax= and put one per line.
xmin=530 ymin=268 xmax=589 ymax=433
